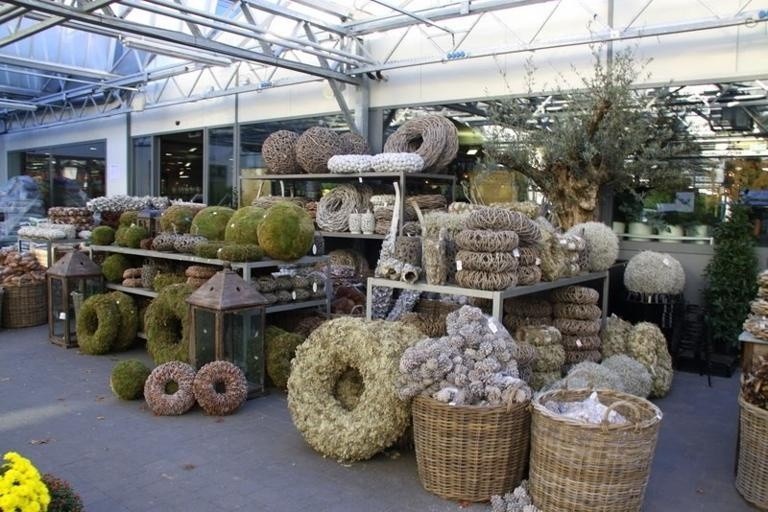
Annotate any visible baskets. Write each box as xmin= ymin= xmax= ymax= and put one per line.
xmin=528 ymin=368 xmax=664 ymax=512
xmin=0 ymin=271 xmax=49 ymax=329
xmin=734 ymin=388 xmax=768 ymax=511
xmin=408 ymin=381 xmax=534 ymax=504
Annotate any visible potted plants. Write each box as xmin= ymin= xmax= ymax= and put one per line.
xmin=611 ymin=181 xmax=722 ymax=245
